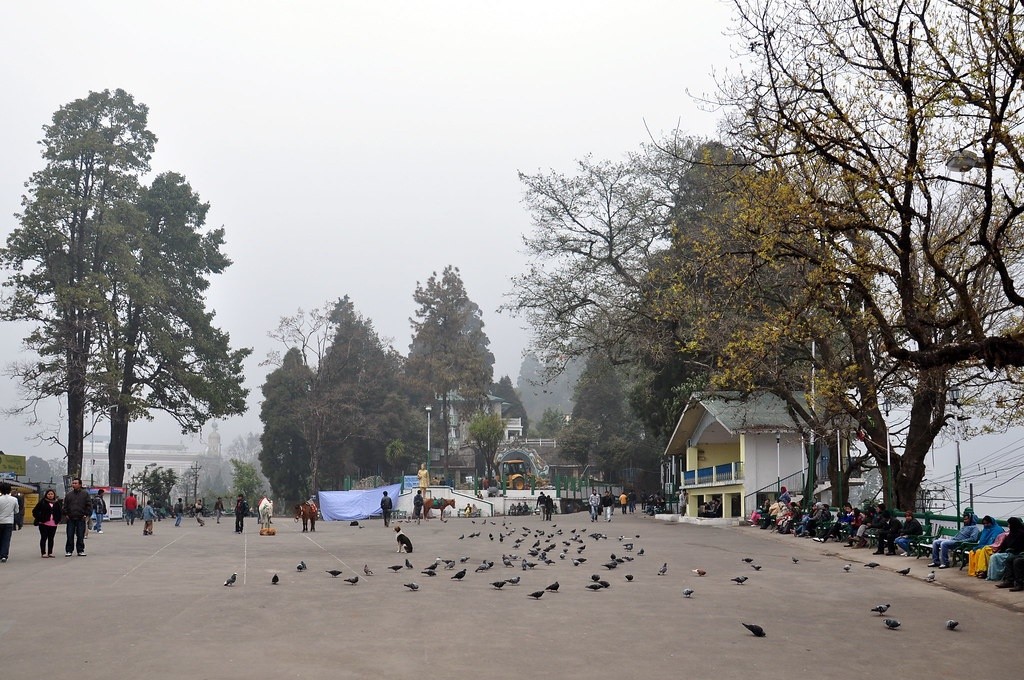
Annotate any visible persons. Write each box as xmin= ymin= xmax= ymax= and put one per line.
xmin=414 ymin=490 xmax=423 ymax=524
xmin=968 ymin=515 xmax=1024 ymax=592
xmin=381 ymin=491 xmax=393 ymax=527
xmin=195 ymin=499 xmax=205 ymax=526
xmin=0 ymin=482 xmax=19 ymax=562
xmin=748 ymin=486 xmax=888 ymax=549
xmin=873 ymin=510 xmax=903 ymax=555
xmin=235 ymin=494 xmax=249 ymax=532
xmin=63 ymin=478 xmax=93 ymax=556
xmin=509 ymin=491 xmax=553 ymax=521
xmin=125 ymin=493 xmax=136 ymax=525
xmin=32 ymin=489 xmax=62 ymax=558
xmin=417 ymin=463 xmax=429 ymax=498
xmin=174 ymin=498 xmax=183 ymax=526
xmin=927 ymin=513 xmax=978 ymax=568
xmin=154 ymin=498 xmax=162 ymax=522
xmin=93 ymin=489 xmax=107 ymax=534
xmin=895 ymin=510 xmax=923 ymax=557
xmin=11 ymin=490 xmax=23 ymax=530
xmin=215 ymin=497 xmax=224 ymax=523
xmin=589 ymin=489 xmax=722 ymax=522
xmin=142 ymin=500 xmax=157 ymax=535
xmin=431 ymin=475 xmax=531 ymax=490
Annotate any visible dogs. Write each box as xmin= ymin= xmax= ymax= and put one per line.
xmin=260 ymin=528 xmax=277 ymax=536
xmin=393 ymin=526 xmax=412 ymax=554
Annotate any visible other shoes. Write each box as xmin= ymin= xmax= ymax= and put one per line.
xmin=2 ymin=558 xmax=7 ymax=561
xmin=608 ymin=519 xmax=611 ymax=522
xmin=65 ymin=552 xmax=72 ymax=556
xmin=417 ymin=518 xmax=420 ymax=525
xmin=995 ymin=581 xmax=1013 ymax=587
xmin=99 ymin=530 xmax=103 ymax=533
xmin=976 ymin=572 xmax=987 ymax=578
xmin=50 ymin=555 xmax=55 ymax=558
xmin=928 ymin=562 xmax=939 ymax=567
xmin=591 ymin=519 xmax=594 ymax=522
xmin=43 ymin=554 xmax=49 ymax=558
xmin=239 ymin=531 xmax=241 ymax=534
xmin=595 ymin=518 xmax=598 ymax=522
xmin=1010 ymin=585 xmax=1022 ymax=591
xmin=900 ymin=552 xmax=908 ymax=556
xmin=873 ymin=551 xmax=883 ymax=554
xmin=911 ymin=552 xmax=916 ymax=556
xmin=790 ymin=530 xmax=868 ymax=549
xmin=78 ymin=552 xmax=86 ymax=556
xmin=886 ymin=552 xmax=895 ymax=555
xmin=604 ymin=519 xmax=607 ymax=521
xmin=84 ymin=535 xmax=87 ymax=538
xmin=939 ymin=564 xmax=949 ymax=569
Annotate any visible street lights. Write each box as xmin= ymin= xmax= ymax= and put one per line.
xmin=425 ymin=404 xmax=433 ymax=477
xmin=774 ymin=429 xmax=782 ymax=501
xmin=882 ymin=396 xmax=893 ymax=512
xmin=832 ymin=415 xmax=841 ymax=511
xmin=797 ymin=427 xmax=806 ymax=513
xmin=949 ymin=384 xmax=962 ymax=530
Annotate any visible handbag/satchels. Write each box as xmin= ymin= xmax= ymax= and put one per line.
xmin=415 ymin=500 xmax=423 ymax=507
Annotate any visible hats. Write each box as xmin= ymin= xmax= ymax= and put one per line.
xmin=905 ymin=510 xmax=913 ymax=517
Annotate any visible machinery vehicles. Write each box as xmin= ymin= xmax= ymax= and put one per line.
xmin=496 ymin=459 xmax=549 ymax=490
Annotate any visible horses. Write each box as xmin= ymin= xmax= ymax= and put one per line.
xmin=422 ymin=498 xmax=455 ymax=520
xmin=295 ymin=503 xmax=318 ymax=532
xmin=258 ymin=497 xmax=273 ymax=529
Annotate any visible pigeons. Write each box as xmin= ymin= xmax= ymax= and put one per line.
xmin=364 ymin=564 xmax=375 ymax=576
xmin=741 ymin=622 xmax=767 ymax=637
xmin=842 ymin=562 xmax=959 ymax=630
xmin=791 ymin=557 xmax=800 ymax=564
xmin=730 ymin=575 xmax=748 ymax=584
xmin=224 ymin=573 xmax=238 ymax=587
xmin=751 ymin=565 xmax=762 ymax=571
xmin=392 ymin=517 xmax=706 ymax=599
xmin=389 ymin=565 xmax=403 ymax=573
xmin=297 ymin=560 xmax=308 ymax=572
xmin=324 ymin=571 xmax=343 ymax=577
xmin=403 ymin=583 xmax=420 ymax=591
xmin=742 ymin=558 xmax=753 ymax=563
xmin=272 ymin=574 xmax=279 ymax=584
xmin=345 ymin=576 xmax=359 ymax=584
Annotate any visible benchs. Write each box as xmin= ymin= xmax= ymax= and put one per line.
xmin=816 ymin=515 xmax=878 ymax=549
xmin=914 ymin=524 xmax=980 ymax=571
xmin=458 ymin=508 xmax=481 ymax=518
